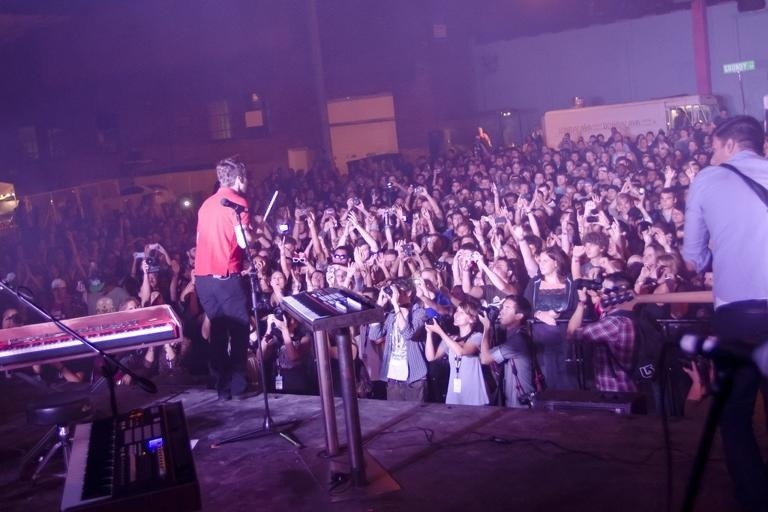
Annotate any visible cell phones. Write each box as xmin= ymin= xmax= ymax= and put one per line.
xmin=149 ymin=244 xmax=159 ymax=249
xmin=135 ymin=253 xmax=145 ymax=257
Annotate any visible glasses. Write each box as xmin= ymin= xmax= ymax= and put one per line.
xmin=334 ymin=255 xmax=347 ymax=259
xmin=293 ymin=258 xmax=305 ymax=262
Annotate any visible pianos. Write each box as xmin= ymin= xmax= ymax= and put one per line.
xmin=1 ymin=303 xmax=185 ymax=370
xmin=61 ymin=400 xmax=201 ymax=511
xmin=280 ymin=286 xmax=385 ymax=333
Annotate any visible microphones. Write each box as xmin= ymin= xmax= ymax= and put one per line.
xmin=221 ymin=198 xmax=249 ymax=213
xmin=0 ymin=272 xmax=16 ymax=290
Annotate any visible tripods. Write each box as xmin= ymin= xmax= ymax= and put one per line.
xmin=215 ymin=276 xmax=306 ymax=450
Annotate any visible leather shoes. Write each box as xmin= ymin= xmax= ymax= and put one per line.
xmin=217 ymin=384 xmax=263 ymax=401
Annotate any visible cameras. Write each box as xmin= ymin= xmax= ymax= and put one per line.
xmin=279 ymin=181 xmax=644 ymax=261
xmin=147 ymin=257 xmax=161 ymax=273
xmin=575 ymin=273 xmax=602 ymax=295
xmin=477 ymin=305 xmax=499 ymax=322
xmin=425 ymin=316 xmax=442 ymax=329
xmin=414 ymin=279 xmax=421 ymax=285
xmin=383 ymin=287 xmax=393 ymax=295
